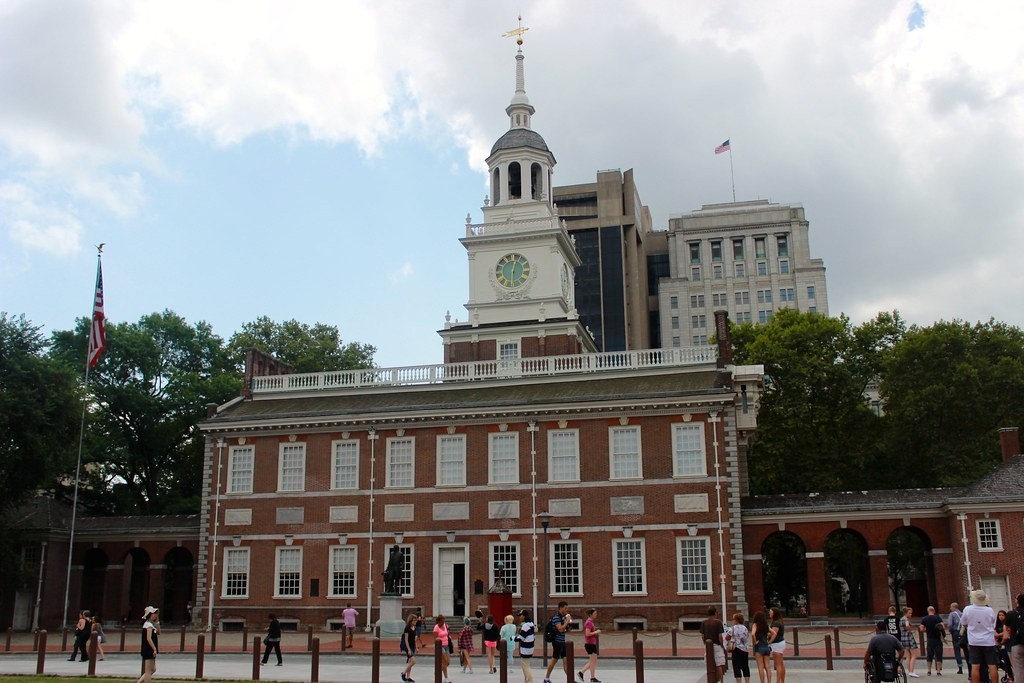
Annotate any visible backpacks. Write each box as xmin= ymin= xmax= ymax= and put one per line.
xmin=544 ymin=615 xmax=562 ymax=642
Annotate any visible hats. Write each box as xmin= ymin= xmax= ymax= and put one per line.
xmin=504 ymin=615 xmax=514 ymax=624
xmin=516 ymin=610 xmax=529 ymax=616
xmin=971 ymin=590 xmax=990 ymax=606
xmin=142 ymin=606 xmax=159 ymax=620
xmin=463 ymin=617 xmax=470 ymax=625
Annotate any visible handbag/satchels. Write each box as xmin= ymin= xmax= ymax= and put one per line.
xmin=448 ymin=636 xmax=454 ymax=653
xmin=958 ymin=628 xmax=967 ymax=647
xmin=101 ymin=635 xmax=105 ymax=643
xmin=400 ymin=634 xmax=406 ymax=649
xmin=264 ymin=636 xmax=269 ymax=645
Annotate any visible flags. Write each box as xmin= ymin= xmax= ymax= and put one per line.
xmin=715 ymin=139 xmax=730 ymax=154
xmin=88 ymin=261 xmax=107 ymax=368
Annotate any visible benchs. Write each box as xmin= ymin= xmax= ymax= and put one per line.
xmin=873 ymin=614 xmax=890 ymax=625
xmin=810 ymin=616 xmax=830 ymax=626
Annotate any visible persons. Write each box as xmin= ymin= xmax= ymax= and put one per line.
xmin=386 ymin=545 xmax=404 ymax=594
xmin=458 ymin=617 xmax=474 ymax=674
xmin=577 ymin=609 xmax=601 ymax=683
xmin=400 ymin=614 xmax=417 ymax=683
xmin=700 ymin=608 xmax=724 ymax=683
xmin=342 ymin=603 xmax=359 ymax=648
xmin=751 ymin=612 xmax=777 ymax=683
xmin=863 ymin=590 xmax=1024 ymax=683
xmin=500 ymin=615 xmax=516 ymax=673
xmin=544 ymin=601 xmax=572 ymax=683
xmin=187 ymin=601 xmax=192 ymax=622
xmin=432 ymin=615 xmax=452 ymax=683
xmin=415 ymin=608 xmax=426 ymax=648
xmin=260 ymin=613 xmax=283 ymax=666
xmin=767 ymin=608 xmax=785 ymax=683
xmin=67 ymin=610 xmax=104 ymax=662
xmin=477 ymin=616 xmax=501 ymax=674
xmin=725 ymin=614 xmax=750 ymax=683
xmin=136 ymin=606 xmax=160 ymax=683
xmin=510 ymin=610 xmax=536 ymax=683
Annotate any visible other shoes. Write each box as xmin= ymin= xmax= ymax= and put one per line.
xmin=406 ymin=678 xmax=415 ymax=682
xmin=493 ymin=667 xmax=497 ymax=673
xmin=543 ymin=678 xmax=551 ymax=683
xmin=466 ymin=670 xmax=474 ymax=674
xmin=444 ymin=678 xmax=451 ymax=683
xmin=908 ymin=672 xmax=973 ymax=680
xmin=401 ymin=672 xmax=406 ymax=681
xmin=460 ymin=670 xmax=466 ymax=673
xmin=590 ymin=677 xmax=601 ymax=683
xmin=576 ymin=671 xmax=584 ymax=681
xmin=489 ymin=671 xmax=493 ymax=674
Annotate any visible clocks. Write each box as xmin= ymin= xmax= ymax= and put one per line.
xmin=494 ymin=252 xmax=532 ymax=290
